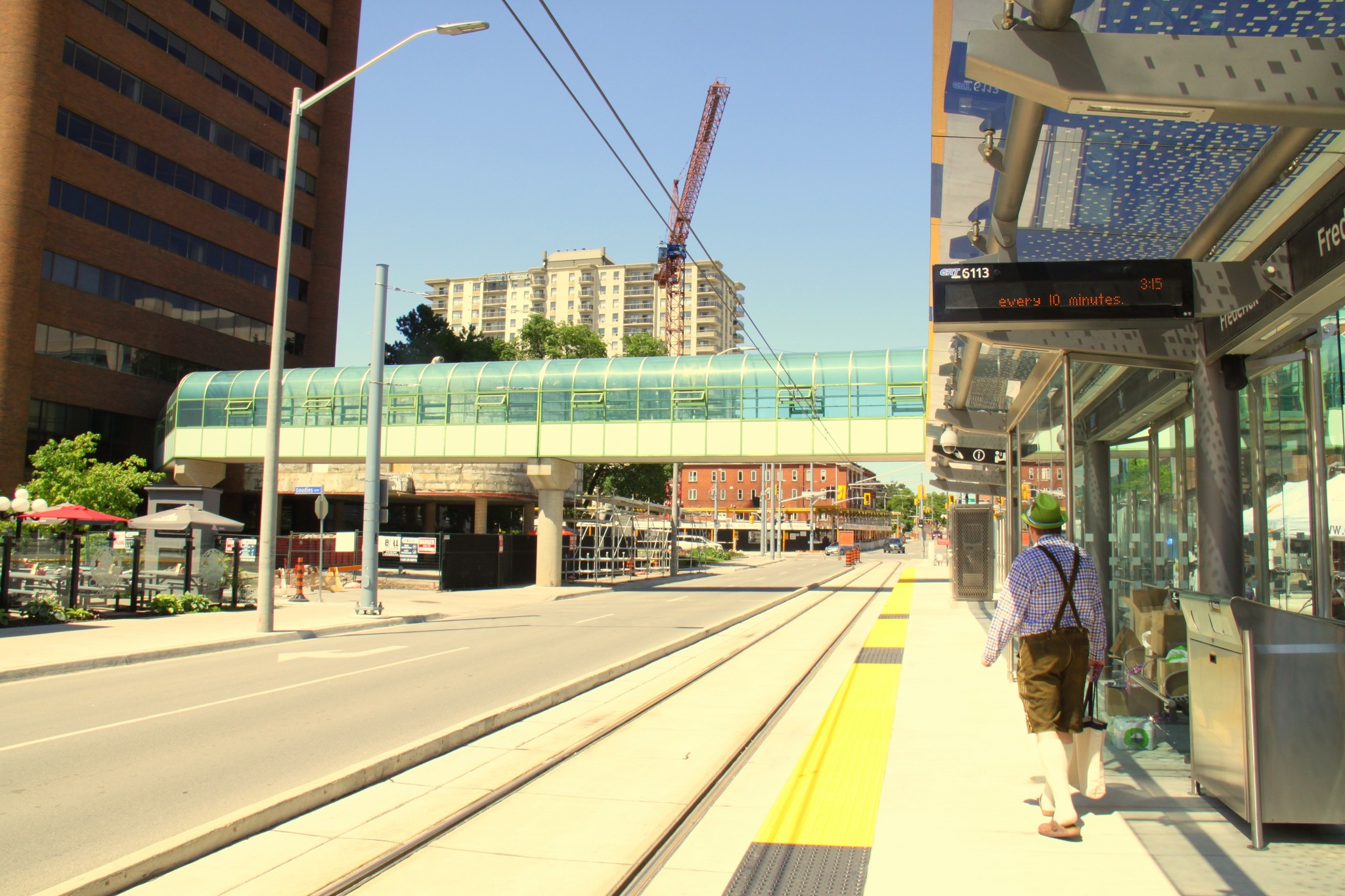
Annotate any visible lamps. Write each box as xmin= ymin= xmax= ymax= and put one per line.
xmin=984 ymin=128 xmax=996 ymax=157
xmin=1002 ymin=0 xmax=1015 ymax=30
xmin=972 ymin=220 xmax=981 ymax=241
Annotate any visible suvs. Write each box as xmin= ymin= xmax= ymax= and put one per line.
xmin=932 ymin=530 xmax=943 ymax=539
xmin=883 ymin=536 xmax=905 ymax=554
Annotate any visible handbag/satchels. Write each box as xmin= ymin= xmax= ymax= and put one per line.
xmin=1067 ymin=679 xmax=1107 ymax=798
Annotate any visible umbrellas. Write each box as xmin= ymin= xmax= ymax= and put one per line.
xmin=11 ymin=504 xmax=133 ymax=566
xmin=128 ymin=501 xmax=246 ymax=593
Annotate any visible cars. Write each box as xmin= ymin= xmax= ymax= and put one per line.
xmin=668 ymin=535 xmax=723 ymax=557
xmin=825 ymin=541 xmax=860 ymax=556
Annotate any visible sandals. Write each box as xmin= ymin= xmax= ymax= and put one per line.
xmin=1039 ymin=793 xmax=1055 ymax=817
xmin=1038 ymin=817 xmax=1080 ymax=838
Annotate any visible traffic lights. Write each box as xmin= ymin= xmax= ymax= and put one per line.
xmin=949 ymin=494 xmax=955 ymax=506
xmin=864 ymin=493 xmax=870 ymax=506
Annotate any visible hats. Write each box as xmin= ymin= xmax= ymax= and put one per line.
xmin=1022 ymin=493 xmax=1068 ymax=529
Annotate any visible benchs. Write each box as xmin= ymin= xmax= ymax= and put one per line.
xmin=9 ymin=581 xmax=208 ymax=609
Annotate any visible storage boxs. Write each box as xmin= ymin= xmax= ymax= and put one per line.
xmin=1099 ymin=589 xmax=1187 ymax=717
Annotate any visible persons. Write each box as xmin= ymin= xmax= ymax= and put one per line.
xmin=983 ymin=493 xmax=1106 ymax=839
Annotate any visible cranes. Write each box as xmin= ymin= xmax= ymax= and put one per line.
xmin=652 ymin=76 xmax=731 ymax=354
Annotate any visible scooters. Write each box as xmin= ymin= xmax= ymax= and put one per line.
xmin=1272 ymin=568 xmax=1345 ymax=621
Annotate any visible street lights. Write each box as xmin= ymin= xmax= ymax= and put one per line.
xmin=0 ymin=487 xmax=47 ymax=571
xmin=251 ymin=19 xmax=491 ymax=622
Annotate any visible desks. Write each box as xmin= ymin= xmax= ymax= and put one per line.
xmin=122 ymin=575 xmax=155 ymax=609
xmin=10 ymin=572 xmax=62 ymax=581
xmin=142 ymin=570 xmax=200 ymax=598
